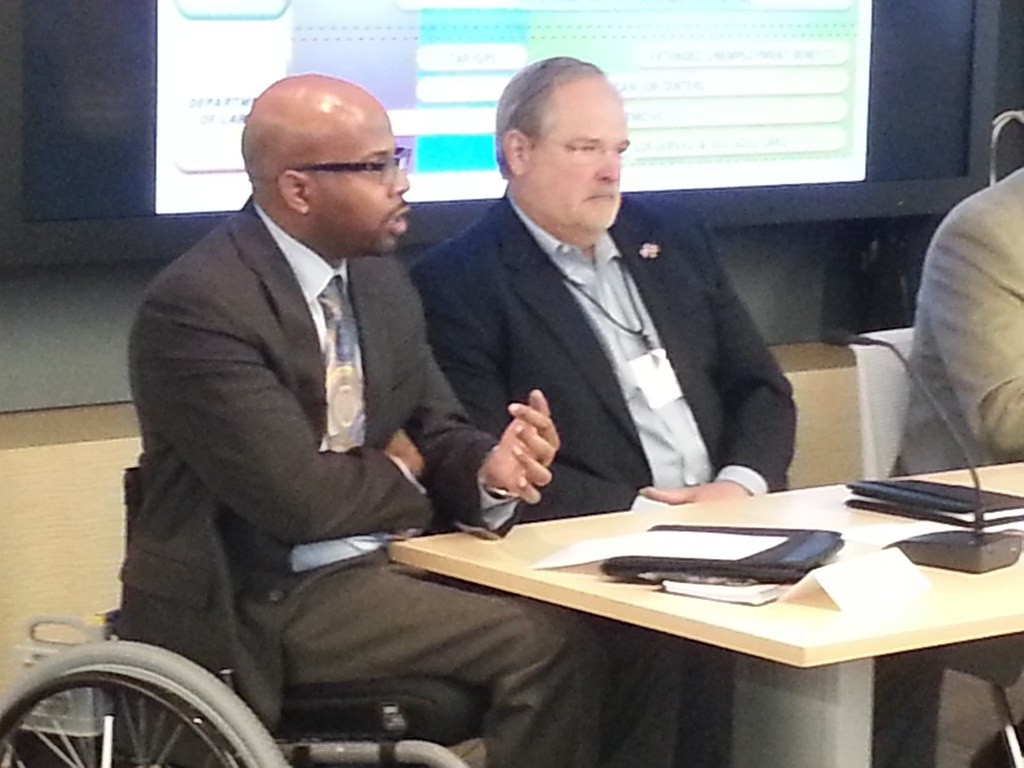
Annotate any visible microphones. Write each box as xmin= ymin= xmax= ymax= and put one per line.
xmin=816 ymin=325 xmax=1023 ymax=574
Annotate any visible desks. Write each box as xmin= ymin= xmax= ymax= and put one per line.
xmin=384 ymin=460 xmax=1024 ymax=768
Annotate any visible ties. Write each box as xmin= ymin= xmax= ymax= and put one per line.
xmin=317 ymin=276 xmax=368 ymax=452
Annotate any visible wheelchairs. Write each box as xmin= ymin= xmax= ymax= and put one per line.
xmin=0 ymin=467 xmax=490 ymax=768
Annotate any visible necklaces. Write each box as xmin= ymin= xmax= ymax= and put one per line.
xmin=558 ymin=256 xmax=656 ymax=353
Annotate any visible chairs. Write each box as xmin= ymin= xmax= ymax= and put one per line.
xmin=848 ymin=328 xmax=1024 ymax=768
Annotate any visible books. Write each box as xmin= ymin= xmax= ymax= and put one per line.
xmin=604 ymin=524 xmax=844 ymax=585
xmin=847 ymin=475 xmax=1024 ymax=530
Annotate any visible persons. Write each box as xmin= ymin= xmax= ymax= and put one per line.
xmin=121 ymin=75 xmax=604 ymax=767
xmin=885 ymin=166 xmax=1024 ymax=477
xmin=410 ymin=55 xmax=798 ymax=767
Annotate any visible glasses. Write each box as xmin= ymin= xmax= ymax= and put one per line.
xmin=299 ymin=145 xmax=415 ymax=185
